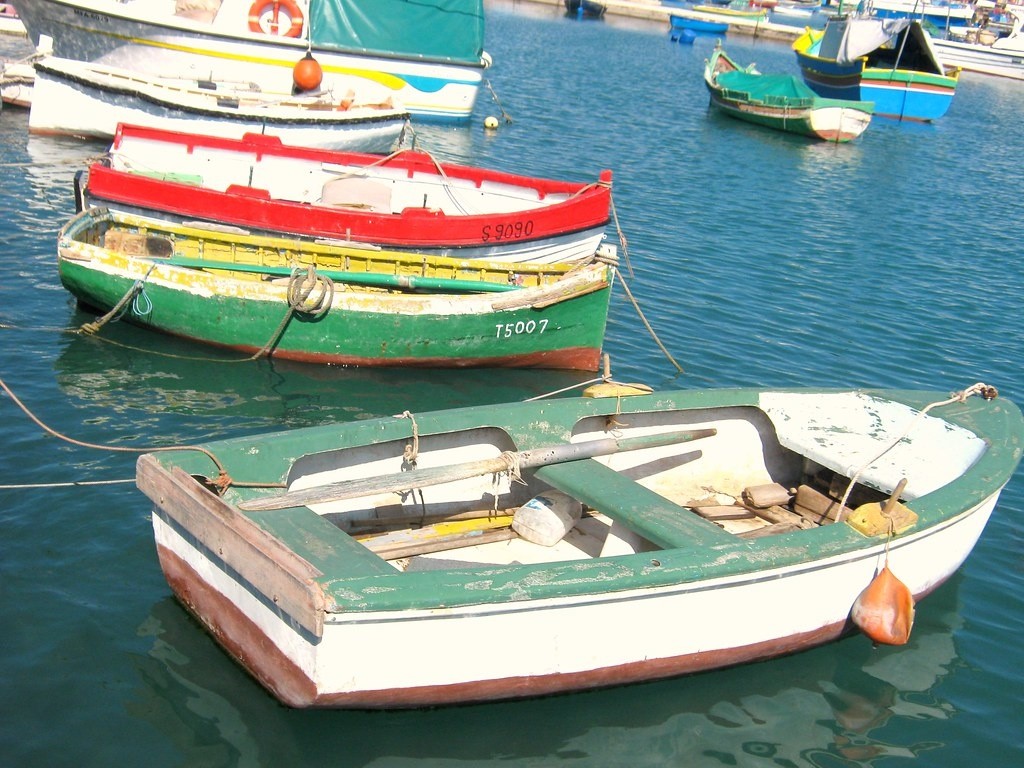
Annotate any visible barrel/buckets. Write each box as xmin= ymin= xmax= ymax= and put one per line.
xmin=512 ymin=489 xmax=582 ymax=546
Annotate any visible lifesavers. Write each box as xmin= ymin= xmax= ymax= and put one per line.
xmin=246 ymin=0 xmax=305 ymax=37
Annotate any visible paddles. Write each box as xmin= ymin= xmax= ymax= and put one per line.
xmin=153 ymin=254 xmax=528 ymax=291
xmin=234 ymin=425 xmax=719 ymax=515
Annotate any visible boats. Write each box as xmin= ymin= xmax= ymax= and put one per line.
xmin=565 ymin=0 xmax=607 ymax=18
xmin=667 ymin=14 xmax=729 ymax=34
xmin=658 ymin=1 xmax=886 ymax=26
xmin=703 ymin=37 xmax=874 ymax=144
xmin=79 ymin=121 xmax=615 ymax=262
xmin=135 ymin=383 xmax=1023 ymax=712
xmin=925 ymin=0 xmax=1024 ymax=83
xmin=23 ymin=34 xmax=420 ymax=151
xmin=1 ymin=1 xmax=514 ymax=130
xmin=53 ymin=207 xmax=618 ymax=375
xmin=792 ymin=1 xmax=965 ymax=126
